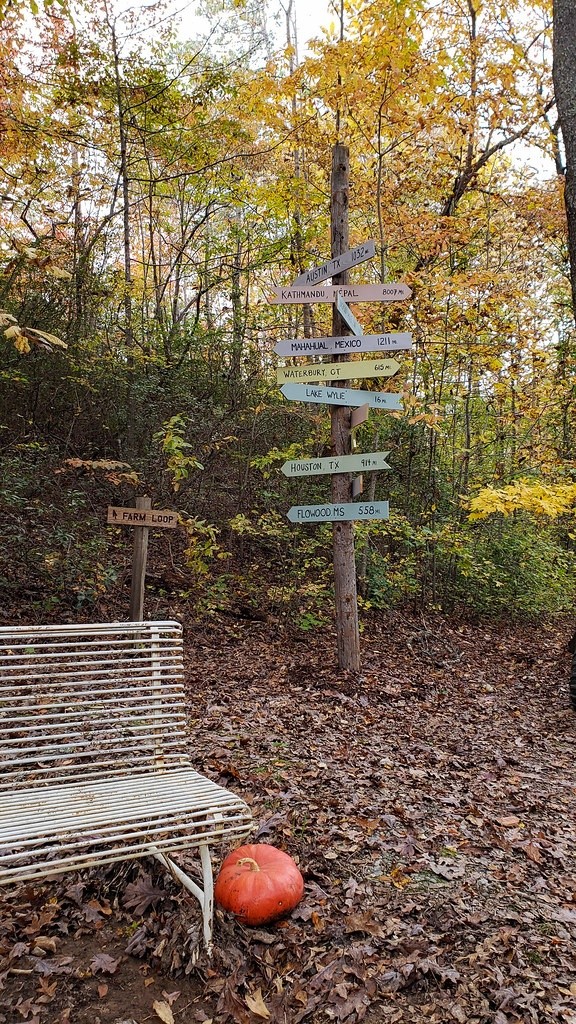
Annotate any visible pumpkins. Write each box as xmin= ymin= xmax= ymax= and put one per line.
xmin=215 ymin=844 xmax=304 ymax=926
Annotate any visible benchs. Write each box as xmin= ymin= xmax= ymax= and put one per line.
xmin=0 ymin=621 xmax=253 ymax=958
